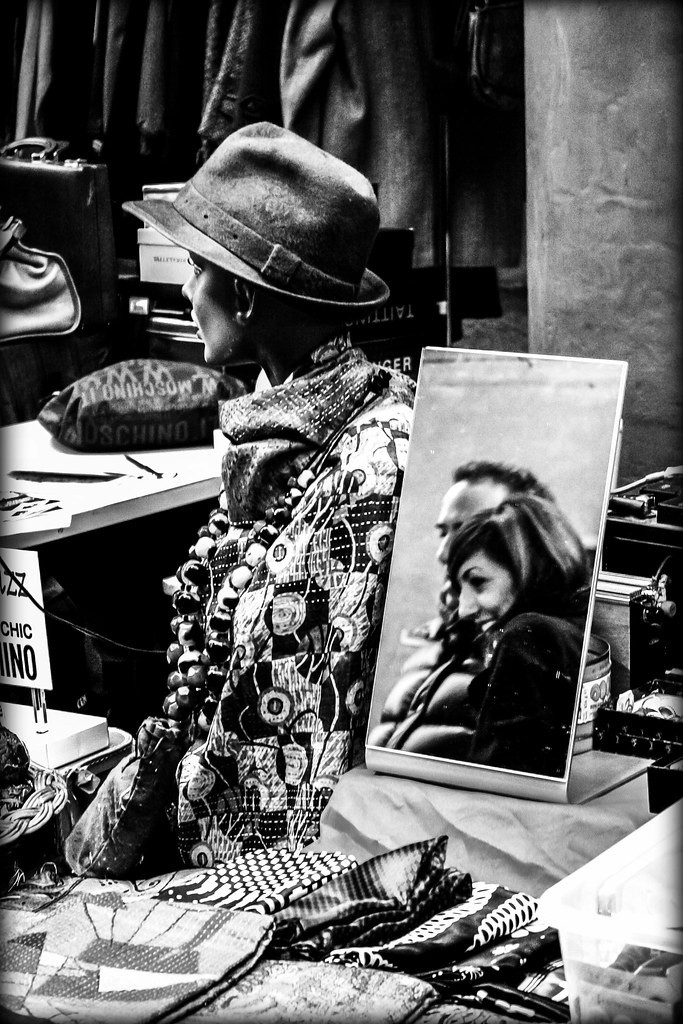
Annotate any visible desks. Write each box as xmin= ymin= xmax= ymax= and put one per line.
xmin=1 ymin=420 xmax=222 ymax=549
xmin=319 ymin=749 xmax=655 ymax=899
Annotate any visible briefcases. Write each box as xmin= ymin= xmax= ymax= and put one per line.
xmin=0 ymin=136 xmax=115 ymax=335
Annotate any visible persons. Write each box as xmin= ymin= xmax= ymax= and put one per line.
xmin=118 ymin=120 xmax=415 ymax=872
xmin=380 ymin=463 xmax=587 ymax=780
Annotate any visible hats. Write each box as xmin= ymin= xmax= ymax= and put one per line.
xmin=122 ymin=121 xmax=391 ymax=307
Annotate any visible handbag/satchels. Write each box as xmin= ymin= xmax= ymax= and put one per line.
xmin=36 ymin=357 xmax=249 ymax=453
xmin=0 ymin=216 xmax=85 ymax=343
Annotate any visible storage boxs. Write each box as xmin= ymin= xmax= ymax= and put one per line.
xmin=535 ymin=800 xmax=683 ymax=1024
xmin=141 ymin=182 xmax=185 ymax=228
xmin=137 ymin=228 xmax=194 ymax=285
